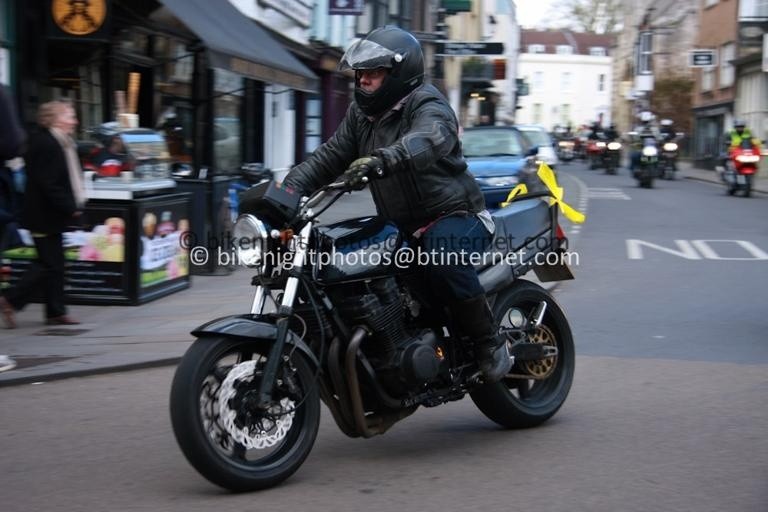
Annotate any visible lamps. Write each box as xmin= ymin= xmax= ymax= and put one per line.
xmin=487 ymin=14 xmax=496 ymax=24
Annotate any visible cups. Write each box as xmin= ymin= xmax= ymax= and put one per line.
xmin=120 ymin=171 xmax=134 ymax=184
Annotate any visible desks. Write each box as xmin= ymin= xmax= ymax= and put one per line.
xmin=0 ymin=189 xmax=193 ymax=308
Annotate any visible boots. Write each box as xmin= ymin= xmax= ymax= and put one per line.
xmin=458 ymin=293 xmax=512 ymax=386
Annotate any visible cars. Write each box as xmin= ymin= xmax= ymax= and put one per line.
xmin=214 ymin=117 xmax=239 ymax=176
xmin=85 ymin=127 xmax=170 ymax=171
xmin=459 ymin=125 xmax=558 ymax=210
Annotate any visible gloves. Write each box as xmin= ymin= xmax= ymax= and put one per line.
xmin=343 ymin=154 xmax=384 ymax=187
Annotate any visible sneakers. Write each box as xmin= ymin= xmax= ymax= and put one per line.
xmin=48 ymin=316 xmax=82 ymax=324
xmin=0 ymin=295 xmax=17 ymax=328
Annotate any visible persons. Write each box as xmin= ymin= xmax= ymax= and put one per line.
xmin=0 ymin=97 xmax=86 ymax=330
xmin=0 ymin=83 xmax=29 ymax=375
xmin=562 ymin=110 xmax=756 ymax=169
xmin=274 ymin=26 xmax=514 ymax=384
xmin=139 ymin=210 xmax=189 ymax=281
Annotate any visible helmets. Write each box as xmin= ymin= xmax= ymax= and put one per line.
xmin=660 ymin=119 xmax=673 ymax=126
xmin=640 ymin=111 xmax=652 ymax=122
xmin=734 ymin=119 xmax=745 ymax=127
xmin=335 ymin=24 xmax=425 ymax=116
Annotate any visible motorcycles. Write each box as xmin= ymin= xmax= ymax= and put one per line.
xmin=716 ymin=140 xmax=763 ymax=197
xmin=170 ymin=167 xmax=574 ymax=492
xmin=556 ymin=132 xmax=684 ymax=188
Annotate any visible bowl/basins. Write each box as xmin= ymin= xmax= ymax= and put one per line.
xmin=118 ymin=113 xmax=139 ymax=128
